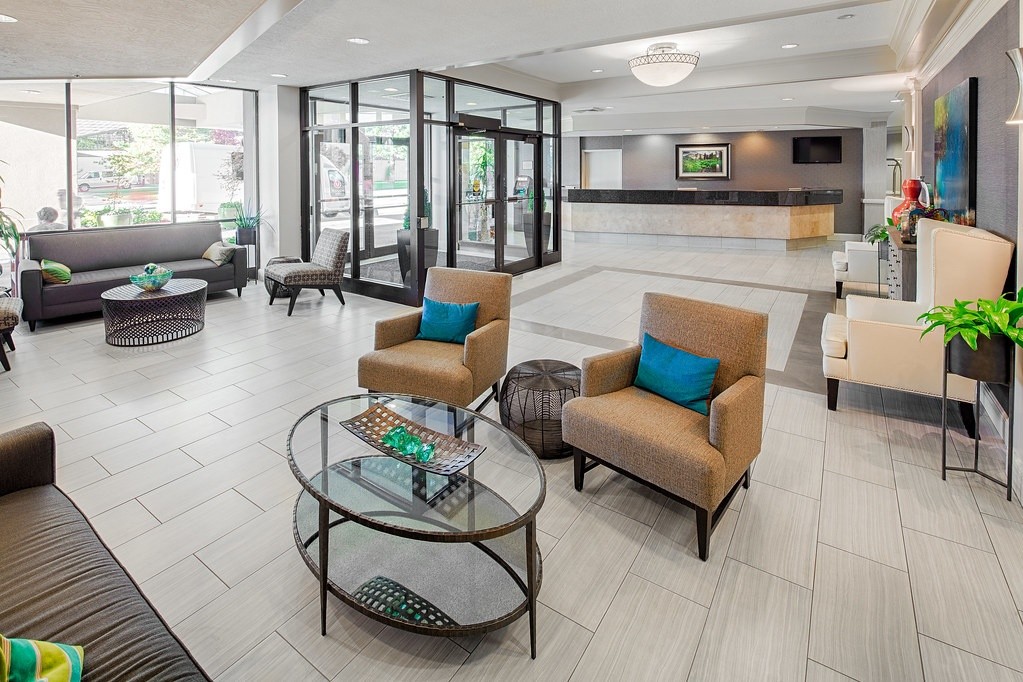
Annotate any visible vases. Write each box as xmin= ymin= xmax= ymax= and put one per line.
xmin=891 ymin=179 xmax=928 ymax=228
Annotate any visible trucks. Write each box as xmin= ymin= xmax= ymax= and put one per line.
xmin=158 ymin=140 xmax=364 ymax=223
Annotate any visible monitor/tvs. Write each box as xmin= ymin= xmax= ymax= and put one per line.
xmin=792 ymin=136 xmax=842 ymax=164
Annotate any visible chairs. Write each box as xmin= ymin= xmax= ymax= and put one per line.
xmin=831 ymin=196 xmax=904 ymax=298
xmin=358 ymin=266 xmax=513 ymax=440
xmin=561 ymin=293 xmax=770 ymax=561
xmin=0 ymin=286 xmax=24 ymax=371
xmin=263 ymin=228 xmax=349 ymax=316
xmin=820 ymin=217 xmax=1015 ymax=439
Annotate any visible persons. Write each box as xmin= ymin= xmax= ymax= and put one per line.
xmin=26 ymin=207 xmax=68 ymax=230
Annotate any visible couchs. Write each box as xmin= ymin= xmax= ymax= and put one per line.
xmin=18 ymin=222 xmax=248 ymax=331
xmin=0 ymin=422 xmax=213 ymax=682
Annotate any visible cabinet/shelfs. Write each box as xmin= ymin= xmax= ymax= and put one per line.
xmin=885 ymin=225 xmax=917 ymax=301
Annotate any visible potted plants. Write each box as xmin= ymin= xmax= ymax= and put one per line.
xmin=96 ymin=204 xmax=133 ymax=226
xmin=915 ymin=287 xmax=1023 ymax=384
xmin=397 ymin=187 xmax=439 ymax=286
xmin=216 ymin=202 xmax=244 ymax=229
xmin=865 ymin=217 xmax=894 ymax=259
xmin=524 ymin=186 xmax=552 ymax=257
xmin=232 ymin=198 xmax=276 ymax=245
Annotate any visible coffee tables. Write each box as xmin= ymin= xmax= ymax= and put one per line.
xmin=285 ymin=393 xmax=546 ymax=660
xmin=266 ymin=257 xmax=303 ymax=298
xmin=100 ymin=278 xmax=208 ymax=347
xmin=499 ymin=359 xmax=581 ymax=459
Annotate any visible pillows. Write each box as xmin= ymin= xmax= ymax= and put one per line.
xmin=416 ymin=297 xmax=481 ymax=344
xmin=202 ymin=241 xmax=236 ymax=267
xmin=0 ymin=633 xmax=85 ymax=682
xmin=41 ymin=259 xmax=72 ymax=284
xmin=633 ymin=333 xmax=721 ymax=416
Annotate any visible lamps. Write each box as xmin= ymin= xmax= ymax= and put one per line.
xmin=1005 ymin=48 xmax=1023 ymax=123
xmin=904 ymin=125 xmax=913 ymax=150
xmin=629 ymin=43 xmax=701 ymax=86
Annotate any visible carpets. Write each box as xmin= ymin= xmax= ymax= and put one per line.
xmin=344 ymin=252 xmax=516 ymax=285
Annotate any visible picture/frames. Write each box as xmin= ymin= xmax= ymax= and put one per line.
xmin=676 ymin=143 xmax=732 ymax=181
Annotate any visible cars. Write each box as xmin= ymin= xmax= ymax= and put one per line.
xmin=78 ymin=167 xmax=139 ymax=192
